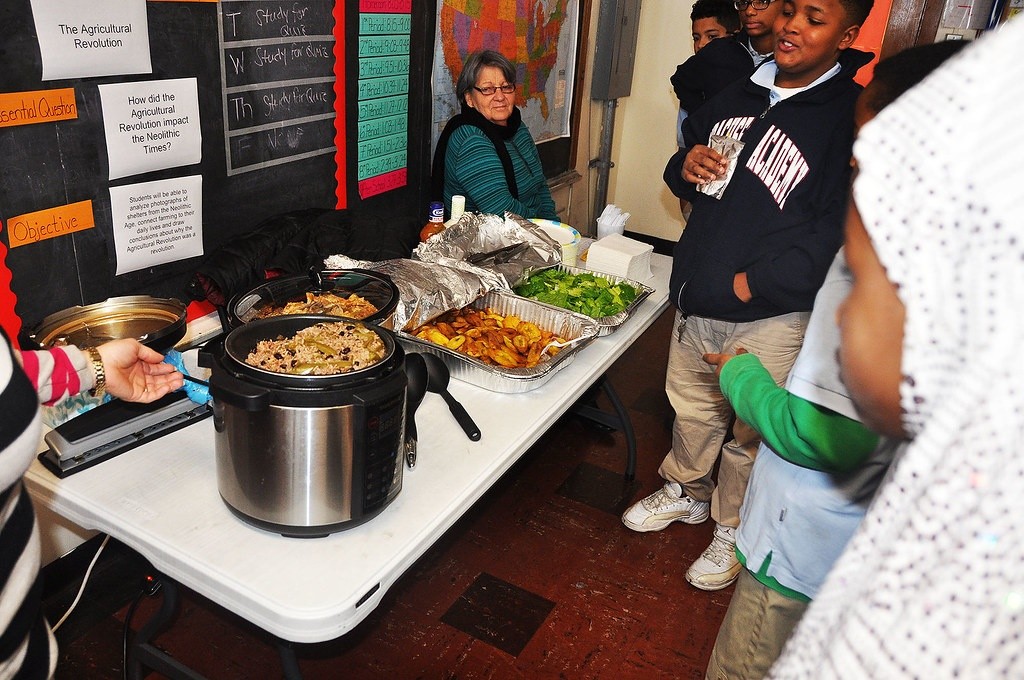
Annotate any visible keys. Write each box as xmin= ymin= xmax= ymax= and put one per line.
xmin=678 ymin=325 xmax=685 ymax=343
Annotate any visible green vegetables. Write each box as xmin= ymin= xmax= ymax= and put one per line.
xmin=514 ymin=269 xmax=638 ymax=317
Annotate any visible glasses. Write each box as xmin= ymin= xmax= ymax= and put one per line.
xmin=733 ymin=0 xmax=776 ymax=11
xmin=467 ymin=78 xmax=517 ymax=95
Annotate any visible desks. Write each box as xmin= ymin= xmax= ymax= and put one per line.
xmin=18 ymin=224 xmax=676 ymax=679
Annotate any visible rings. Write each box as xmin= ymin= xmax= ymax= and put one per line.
xmin=145 ymin=386 xmax=148 ymax=392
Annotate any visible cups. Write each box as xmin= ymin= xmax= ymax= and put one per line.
xmin=596 ymin=218 xmax=626 ymax=240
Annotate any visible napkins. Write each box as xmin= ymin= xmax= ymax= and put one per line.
xmin=586 ymin=232 xmax=654 ymax=283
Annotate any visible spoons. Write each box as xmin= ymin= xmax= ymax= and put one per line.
xmin=404 ymin=352 xmax=430 ymax=467
xmin=421 ymin=352 xmax=482 ymax=442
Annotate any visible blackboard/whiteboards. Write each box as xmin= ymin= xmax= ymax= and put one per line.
xmin=0 ymin=0 xmax=592 ymax=350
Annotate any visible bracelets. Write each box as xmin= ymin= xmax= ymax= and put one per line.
xmin=80 ymin=346 xmax=106 ymax=397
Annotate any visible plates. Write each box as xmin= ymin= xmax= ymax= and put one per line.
xmin=526 ymin=218 xmax=581 ymax=267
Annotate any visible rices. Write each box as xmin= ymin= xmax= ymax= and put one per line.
xmin=245 ymin=322 xmax=385 ymax=375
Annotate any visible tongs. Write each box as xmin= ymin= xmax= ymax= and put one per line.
xmin=465 ymin=241 xmax=530 ymax=266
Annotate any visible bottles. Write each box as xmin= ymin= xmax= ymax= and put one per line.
xmin=420 ymin=201 xmax=447 ymax=243
xmin=443 ymin=196 xmax=466 ymax=229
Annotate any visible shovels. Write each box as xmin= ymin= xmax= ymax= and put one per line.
xmin=404 ymin=353 xmax=431 ymax=469
xmin=419 ymin=352 xmax=482 ymax=442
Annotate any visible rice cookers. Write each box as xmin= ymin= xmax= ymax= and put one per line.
xmin=198 ymin=313 xmax=409 ymax=538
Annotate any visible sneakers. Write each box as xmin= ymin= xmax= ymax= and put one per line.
xmin=686 ymin=520 xmax=744 ymax=592
xmin=621 ymin=482 xmax=712 ymax=533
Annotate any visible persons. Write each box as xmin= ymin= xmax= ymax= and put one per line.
xmin=620 ymin=0 xmax=876 ymax=591
xmin=702 ymin=39 xmax=974 ymax=679
xmin=763 ymin=13 xmax=1024 ymax=680
xmin=430 ymin=50 xmax=560 ymax=221
xmin=0 ymin=328 xmax=185 ymax=680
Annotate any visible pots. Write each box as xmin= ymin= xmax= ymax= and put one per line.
xmin=226 ymin=266 xmax=400 ymax=330
